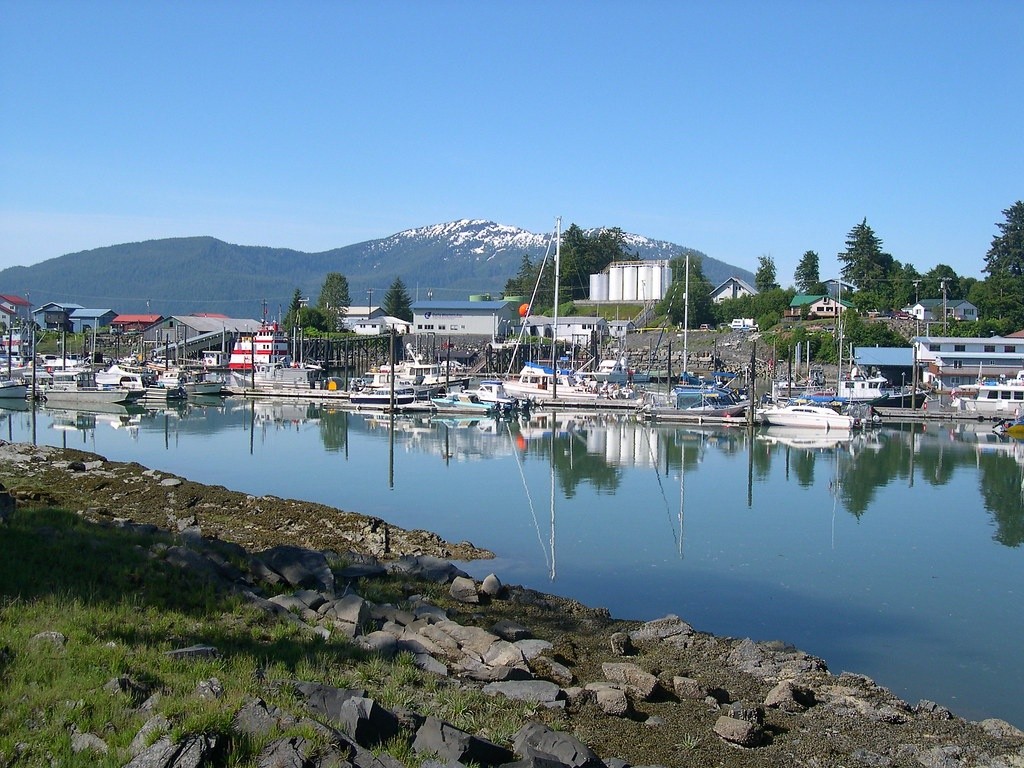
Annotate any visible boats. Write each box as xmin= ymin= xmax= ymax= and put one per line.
xmin=758 ymin=335 xmax=859 ymax=430
xmin=432 ymin=392 xmax=517 ymax=415
xmin=206 ymin=297 xmax=308 ymax=374
xmin=229 ymin=399 xmax=311 ymax=444
xmin=427 ymin=413 xmax=513 ymax=435
xmin=821 ymin=342 xmax=929 ymax=410
xmin=462 ymin=380 xmax=518 ymax=405
xmin=642 ymin=340 xmax=751 ymax=417
xmin=344 ymin=342 xmax=470 ymax=404
xmin=0 ymin=397 xmax=225 ymax=450
xmin=950 ymin=362 xmax=1024 ymax=413
xmin=759 ymin=423 xmax=873 ymax=453
xmin=0 ymin=315 xmax=226 ymax=403
xmin=223 ymin=361 xmax=349 ymax=402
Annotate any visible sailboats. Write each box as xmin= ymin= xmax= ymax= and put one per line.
xmin=501 ymin=217 xmax=654 ymax=409
xmin=638 ymin=417 xmax=745 ymax=560
xmin=638 ymin=254 xmax=727 ymax=406
xmin=589 ymin=305 xmax=648 ymax=382
xmin=488 ymin=404 xmax=612 ymax=583
